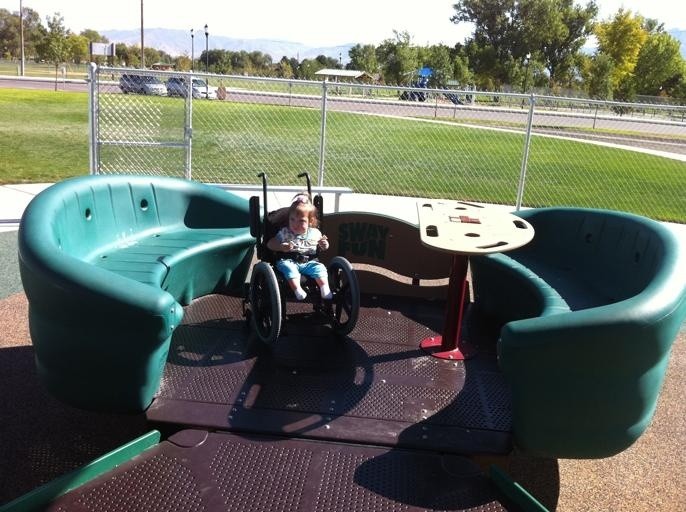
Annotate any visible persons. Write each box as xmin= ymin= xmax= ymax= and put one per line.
xmin=267 ymin=195 xmax=334 ymax=301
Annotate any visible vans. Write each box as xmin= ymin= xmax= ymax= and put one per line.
xmin=167 ymin=78 xmax=216 ymax=100
xmin=119 ymin=74 xmax=166 ymax=96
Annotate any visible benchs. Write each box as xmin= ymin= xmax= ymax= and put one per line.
xmin=474 ymin=207 xmax=686 ymax=460
xmin=17 ymin=173 xmax=266 ymax=416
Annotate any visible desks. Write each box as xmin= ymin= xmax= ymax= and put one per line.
xmin=417 ymin=198 xmax=536 ymax=360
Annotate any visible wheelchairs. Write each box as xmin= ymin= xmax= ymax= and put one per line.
xmin=246 ymin=169 xmax=361 ymax=345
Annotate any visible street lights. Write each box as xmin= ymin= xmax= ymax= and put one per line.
xmin=190 ymin=28 xmax=195 ymax=72
xmin=521 ymin=51 xmax=532 ymax=109
xmin=204 ymin=24 xmax=210 ymax=97
xmin=338 ymin=52 xmax=343 ymax=69
